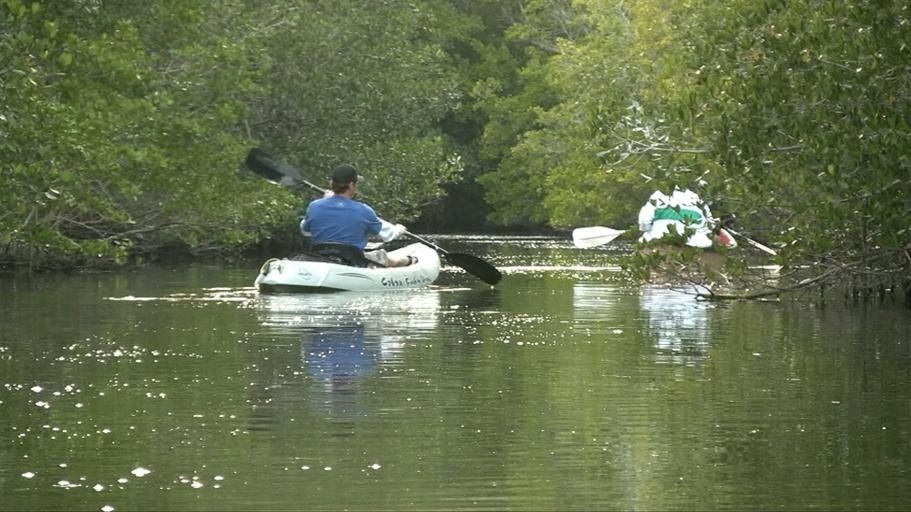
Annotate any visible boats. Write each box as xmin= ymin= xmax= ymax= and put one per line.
xmin=254 ymin=240 xmax=442 ymax=292
xmin=638 ymin=218 xmax=738 ymax=249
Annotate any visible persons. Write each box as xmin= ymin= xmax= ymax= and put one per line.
xmin=641 ymin=288 xmax=712 ymax=368
xmin=297 ymin=163 xmax=420 ymax=268
xmin=307 ymin=321 xmax=383 ymax=416
xmin=637 ymin=178 xmax=739 ymax=252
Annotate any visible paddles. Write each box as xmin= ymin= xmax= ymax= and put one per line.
xmin=720 ymin=222 xmax=775 ymax=256
xmin=571 ymin=224 xmax=636 ymax=250
xmin=246 ymin=147 xmax=502 ymax=284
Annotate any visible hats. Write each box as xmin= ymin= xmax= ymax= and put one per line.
xmin=333 ymin=164 xmax=365 ymax=183
